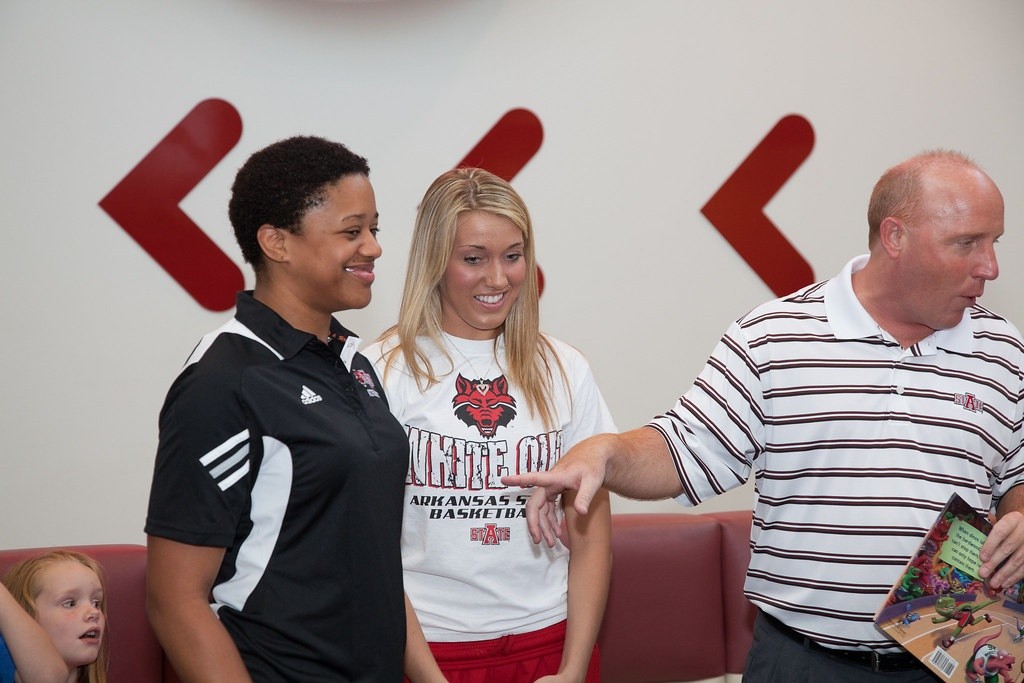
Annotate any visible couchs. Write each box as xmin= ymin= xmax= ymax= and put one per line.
xmin=0 ymin=511 xmax=755 ymax=683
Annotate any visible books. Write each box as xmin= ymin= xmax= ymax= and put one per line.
xmin=871 ymin=492 xmax=1024 ymax=683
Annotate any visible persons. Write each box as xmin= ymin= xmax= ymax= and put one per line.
xmin=0 ymin=549 xmax=107 ymax=683
xmin=500 ymin=150 xmax=1024 ymax=683
xmin=144 ymin=136 xmax=409 ymax=683
xmin=357 ymin=167 xmax=618 ymax=683
